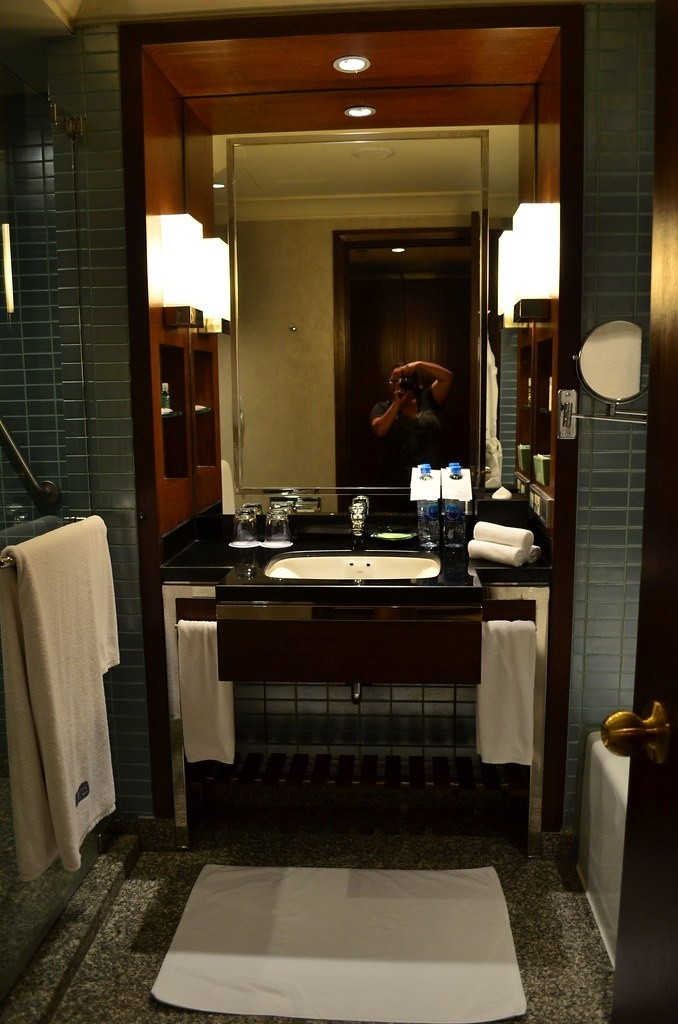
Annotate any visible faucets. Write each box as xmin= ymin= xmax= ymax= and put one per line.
xmin=347 ymin=500 xmax=369 ymax=541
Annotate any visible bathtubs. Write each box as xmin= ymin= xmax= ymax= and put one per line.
xmin=575 ymin=729 xmax=630 ymax=968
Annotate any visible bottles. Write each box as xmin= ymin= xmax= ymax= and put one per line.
xmin=420 ymin=464 xmax=430 ymax=465
xmin=442 ymin=464 xmax=467 ymax=549
xmin=448 ymin=463 xmax=460 ymax=468
xmin=417 ymin=465 xmax=440 ymax=549
xmin=161 ymin=383 xmax=170 ymax=409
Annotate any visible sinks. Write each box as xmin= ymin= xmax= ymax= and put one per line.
xmin=264 ymin=550 xmax=440 ymax=583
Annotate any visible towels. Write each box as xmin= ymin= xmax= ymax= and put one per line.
xmin=177 ymin=620 xmax=236 ymax=766
xmin=468 ymin=520 xmax=542 ymax=567
xmin=475 ymin=618 xmax=536 ymax=768
xmin=0 ymin=513 xmax=120 ymax=884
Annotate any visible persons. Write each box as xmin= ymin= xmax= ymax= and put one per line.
xmin=367 ymin=360 xmax=454 ymax=487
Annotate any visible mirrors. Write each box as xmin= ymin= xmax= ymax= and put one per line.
xmin=177 ymin=83 xmax=540 ymax=514
xmin=224 ymin=132 xmax=494 ymax=512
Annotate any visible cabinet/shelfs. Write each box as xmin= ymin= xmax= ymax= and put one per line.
xmin=163 ymin=580 xmax=548 ymax=858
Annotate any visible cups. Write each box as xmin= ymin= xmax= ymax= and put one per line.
xmin=243 ymin=502 xmax=263 ymax=515
xmin=263 ymin=510 xmax=290 ymax=544
xmin=268 ymin=502 xmax=294 ymax=515
xmin=232 ymin=508 xmax=258 ymax=543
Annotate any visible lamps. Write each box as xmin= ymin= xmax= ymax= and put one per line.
xmin=497 ymin=231 xmax=530 ymax=329
xmin=513 ymin=202 xmax=560 ymax=324
xmin=145 ymin=214 xmax=206 ymax=330
xmin=199 ymin=238 xmax=230 ymax=335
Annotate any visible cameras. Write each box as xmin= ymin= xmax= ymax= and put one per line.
xmin=399 ymin=373 xmax=413 ymax=391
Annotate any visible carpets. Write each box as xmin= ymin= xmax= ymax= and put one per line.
xmin=150 ymin=864 xmax=526 ymax=1024
